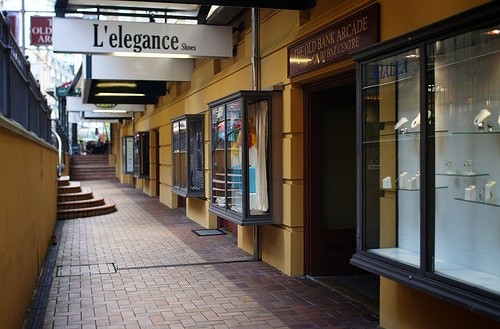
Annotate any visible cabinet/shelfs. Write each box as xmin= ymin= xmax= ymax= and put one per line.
xmin=377 ymin=129 xmax=500 ymax=208
